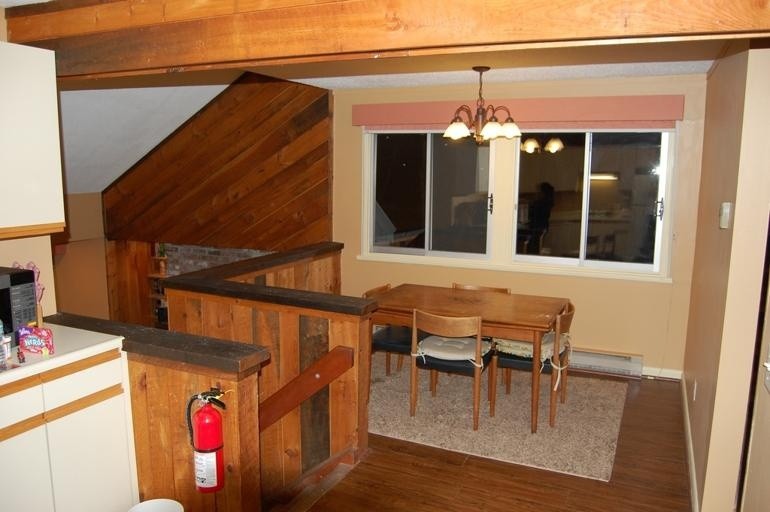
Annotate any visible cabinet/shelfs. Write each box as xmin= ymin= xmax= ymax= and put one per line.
xmin=0 ymin=344 xmax=139 ymax=510
xmin=149 ymin=269 xmax=169 ymax=326
xmin=0 ymin=41 xmax=66 ymax=238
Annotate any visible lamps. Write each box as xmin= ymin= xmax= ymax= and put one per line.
xmin=520 ymin=134 xmax=563 ymax=154
xmin=442 ymin=66 xmax=522 ymax=144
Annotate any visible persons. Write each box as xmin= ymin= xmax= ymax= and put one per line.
xmin=524 ymin=181 xmax=556 ymax=254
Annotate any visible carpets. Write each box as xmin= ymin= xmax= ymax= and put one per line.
xmin=366 ymin=352 xmax=627 ymax=483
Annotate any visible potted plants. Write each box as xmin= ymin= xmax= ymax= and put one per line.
xmin=367 ymin=283 xmax=570 ymax=434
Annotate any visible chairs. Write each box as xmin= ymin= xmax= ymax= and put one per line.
xmin=366 ymin=283 xmax=429 ymax=398
xmin=491 ymin=303 xmax=576 ymax=433
xmin=443 ymin=282 xmax=509 ymax=385
xmin=408 ymin=304 xmax=495 ymax=431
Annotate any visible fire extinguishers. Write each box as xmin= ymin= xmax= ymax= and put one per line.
xmin=188 ymin=390 xmax=228 ymax=494
